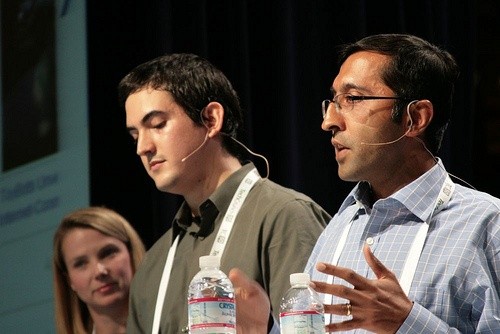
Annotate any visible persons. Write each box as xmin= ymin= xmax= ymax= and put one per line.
xmin=194 ymin=32 xmax=500 ymax=333
xmin=123 ymin=54 xmax=336 ymax=334
xmin=51 ymin=206 xmax=146 ymax=334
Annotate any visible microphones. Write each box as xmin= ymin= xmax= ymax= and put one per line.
xmin=182 ymin=129 xmax=208 ymax=163
xmin=360 ymin=127 xmax=410 ymax=146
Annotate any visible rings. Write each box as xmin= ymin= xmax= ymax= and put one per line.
xmin=345 ymin=303 xmax=352 ymax=316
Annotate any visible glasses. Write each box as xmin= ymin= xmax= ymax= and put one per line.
xmin=321 ymin=90 xmax=406 ymax=118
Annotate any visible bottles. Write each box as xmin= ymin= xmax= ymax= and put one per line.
xmin=187 ymin=254 xmax=237 ymax=334
xmin=280 ymin=271 xmax=327 ymax=334
